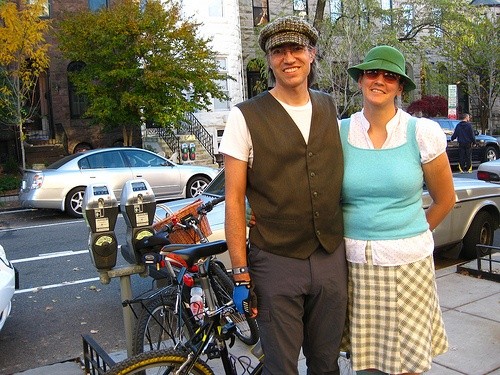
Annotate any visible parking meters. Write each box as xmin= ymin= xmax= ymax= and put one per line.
xmin=83 ymin=179 xmax=156 ymax=357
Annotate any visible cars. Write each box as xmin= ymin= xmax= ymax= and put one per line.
xmin=147 ymin=172 xmax=500 ymax=301
xmin=19 ymin=148 xmax=222 ymax=217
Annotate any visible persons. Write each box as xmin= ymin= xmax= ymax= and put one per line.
xmin=218 ymin=15 xmax=348 ymax=375
xmin=246 ymin=45 xmax=456 ymax=375
xmin=451 ymin=113 xmax=477 ymax=173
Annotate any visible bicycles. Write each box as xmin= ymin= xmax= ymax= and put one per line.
xmin=104 ymin=194 xmax=359 ymax=375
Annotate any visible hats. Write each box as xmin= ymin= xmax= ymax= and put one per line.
xmin=347 ymin=46 xmax=416 ymax=93
xmin=258 ymin=16 xmax=318 ymax=53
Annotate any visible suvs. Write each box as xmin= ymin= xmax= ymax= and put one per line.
xmin=427 ymin=117 xmax=500 ymax=164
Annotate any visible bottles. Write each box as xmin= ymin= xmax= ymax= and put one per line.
xmin=190 ymin=285 xmax=203 ymax=320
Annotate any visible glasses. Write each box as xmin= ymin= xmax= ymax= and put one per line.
xmin=269 ymin=45 xmax=312 ymax=56
xmin=363 ymin=70 xmax=401 ymax=83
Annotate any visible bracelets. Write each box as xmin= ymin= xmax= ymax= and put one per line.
xmin=232 ymin=265 xmax=249 ymax=274
